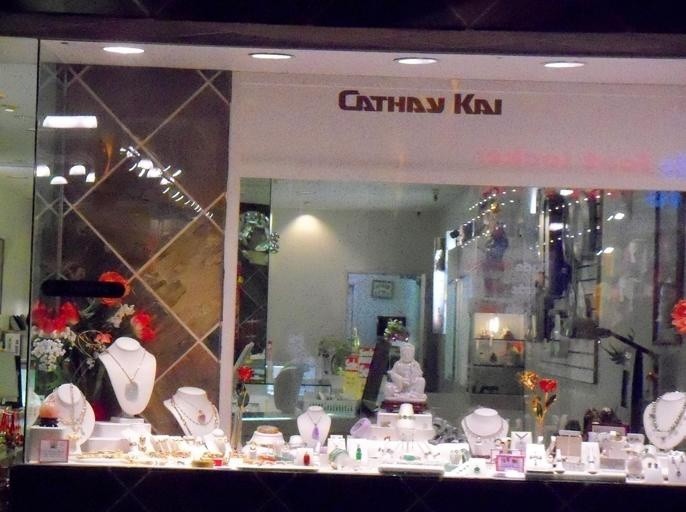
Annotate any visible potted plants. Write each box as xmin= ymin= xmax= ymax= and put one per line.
xmin=383 ymin=318 xmax=409 ymax=342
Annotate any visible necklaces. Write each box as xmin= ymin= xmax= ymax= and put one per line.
xmin=247 ymin=393 xmax=686 ymax=481
xmin=169 ymin=394 xmax=233 ymax=464
xmin=50 ymin=388 xmax=87 ymax=440
xmin=105 ymin=348 xmax=146 ymax=399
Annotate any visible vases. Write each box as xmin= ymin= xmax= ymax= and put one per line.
xmin=229 ymin=405 xmax=244 ymax=451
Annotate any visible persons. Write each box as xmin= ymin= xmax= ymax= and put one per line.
xmin=385 ymin=341 xmax=426 ymax=399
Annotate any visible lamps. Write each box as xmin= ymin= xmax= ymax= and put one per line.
xmin=560 ymin=317 xmax=660 ymax=435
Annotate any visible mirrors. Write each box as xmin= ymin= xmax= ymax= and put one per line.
xmin=230 ymin=176 xmax=686 ymax=447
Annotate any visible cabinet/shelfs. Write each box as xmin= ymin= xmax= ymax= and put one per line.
xmin=466 ymin=311 xmax=528 ymax=396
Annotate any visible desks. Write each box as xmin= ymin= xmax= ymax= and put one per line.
xmin=6 ymin=455 xmax=686 ymax=512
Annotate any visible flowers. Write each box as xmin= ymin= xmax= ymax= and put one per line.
xmin=513 ymin=370 xmax=558 ymax=437
xmin=670 ymin=298 xmax=686 ymax=335
xmin=26 ymin=271 xmax=161 ymax=401
xmin=234 ymin=365 xmax=256 ymax=410
